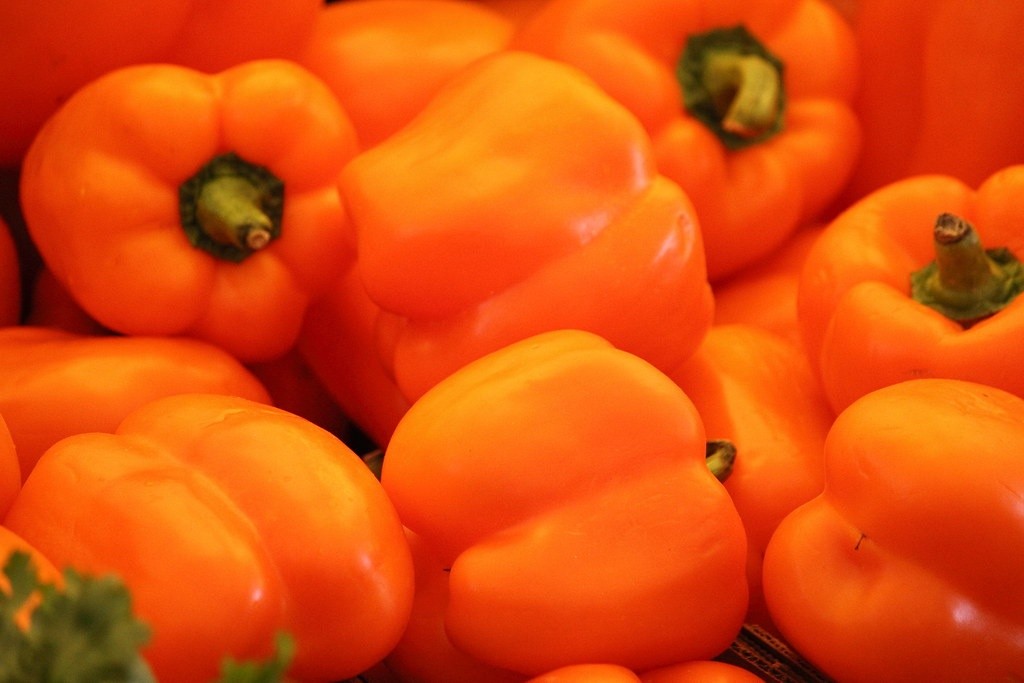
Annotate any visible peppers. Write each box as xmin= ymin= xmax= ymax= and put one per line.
xmin=0 ymin=0 xmax=1024 ymax=683
